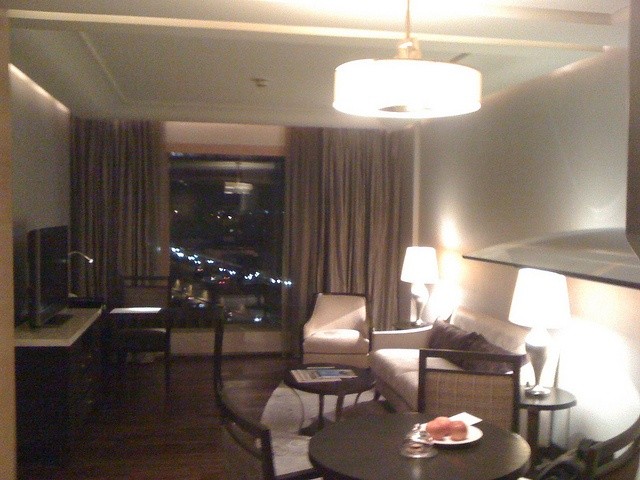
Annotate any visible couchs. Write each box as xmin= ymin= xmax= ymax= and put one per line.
xmin=298 ymin=291 xmax=372 ymax=376
xmin=369 ymin=317 xmax=536 ymax=416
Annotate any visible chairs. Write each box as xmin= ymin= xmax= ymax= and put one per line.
xmin=415 ymin=347 xmax=520 ymax=434
xmin=586 ymin=414 xmax=640 ymax=480
xmin=104 ymin=274 xmax=174 ymax=394
xmin=214 ymin=381 xmax=324 ymax=480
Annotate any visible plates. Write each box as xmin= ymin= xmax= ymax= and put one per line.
xmin=413 ymin=422 xmax=483 ymax=445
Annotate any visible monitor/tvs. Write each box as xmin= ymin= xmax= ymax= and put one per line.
xmin=28 ymin=224 xmax=70 ymax=329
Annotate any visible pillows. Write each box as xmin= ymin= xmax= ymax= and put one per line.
xmin=426 ymin=318 xmax=476 ymax=361
xmin=461 ymin=341 xmax=519 ymax=375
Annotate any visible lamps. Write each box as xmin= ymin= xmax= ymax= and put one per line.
xmin=506 ymin=267 xmax=573 ymax=398
xmin=222 ymin=179 xmax=257 ymax=197
xmin=332 ymin=38 xmax=483 ymax=123
xmin=399 ymin=246 xmax=440 ymax=326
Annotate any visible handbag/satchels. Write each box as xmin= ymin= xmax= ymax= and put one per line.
xmin=537 ymin=439 xmax=613 ymax=480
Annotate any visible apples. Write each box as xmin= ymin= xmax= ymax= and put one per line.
xmin=436 ymin=417 xmax=453 ymax=435
xmin=426 ymin=421 xmax=442 ymax=441
xmin=450 ymin=421 xmax=467 ymax=441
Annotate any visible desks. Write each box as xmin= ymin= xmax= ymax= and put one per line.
xmin=392 ymin=320 xmax=426 ymax=330
xmin=520 ymin=387 xmax=576 ymax=462
xmin=99 ymin=304 xmax=226 ymax=395
xmin=13 ymin=300 xmax=107 ymax=464
xmin=308 ymin=413 xmax=532 ymax=480
